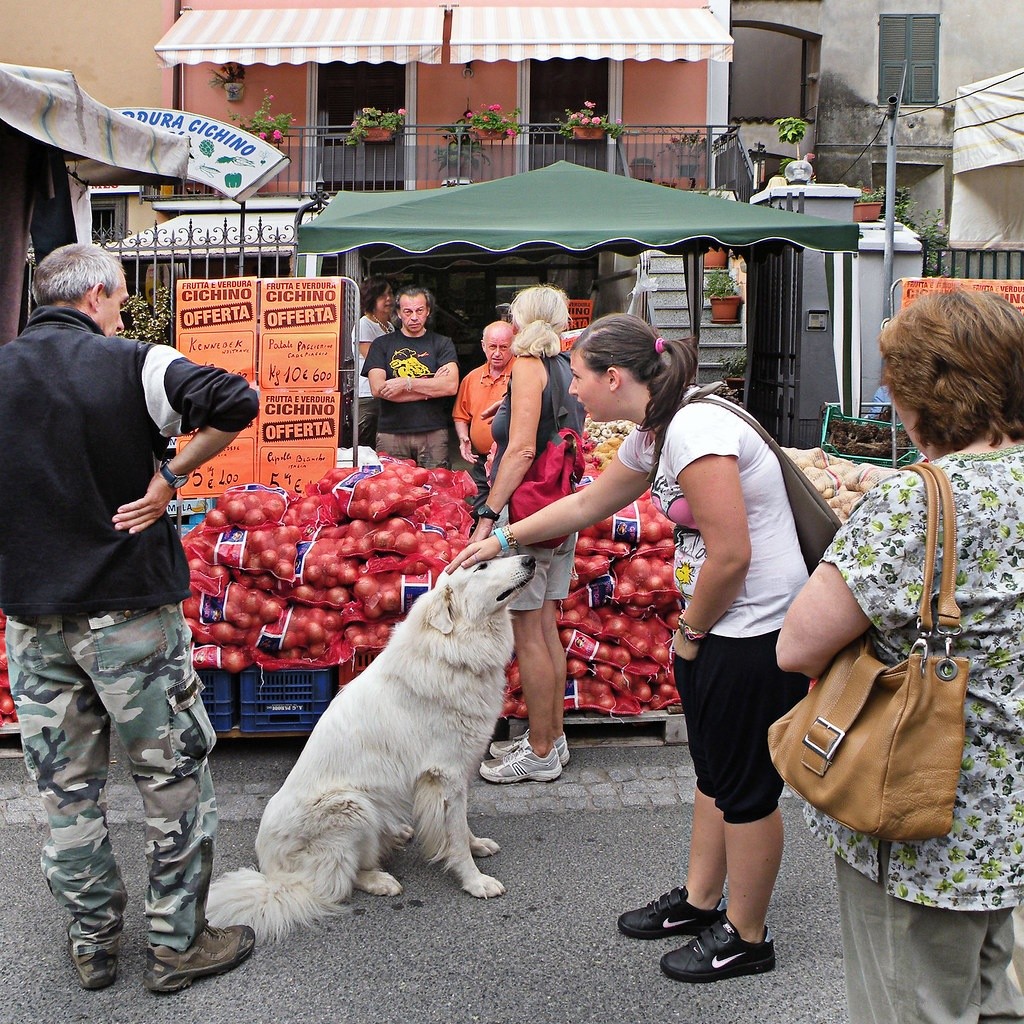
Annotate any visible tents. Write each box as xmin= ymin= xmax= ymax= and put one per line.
xmin=293 ymin=160 xmax=860 ymax=456
xmin=0 ymin=61 xmax=189 ymax=349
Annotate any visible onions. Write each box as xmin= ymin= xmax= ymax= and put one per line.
xmin=178 ymin=459 xmax=682 ymax=712
xmin=0 ymin=610 xmax=17 ymax=726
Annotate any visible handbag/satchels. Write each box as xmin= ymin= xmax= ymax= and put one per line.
xmin=767 ymin=462 xmax=970 ymax=842
xmin=775 ymin=452 xmax=843 ymax=576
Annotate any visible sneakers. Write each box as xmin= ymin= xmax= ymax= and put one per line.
xmin=618 ymin=885 xmax=727 ymax=940
xmin=479 ymin=738 xmax=562 ymax=782
xmin=660 ymin=913 xmax=775 ymax=984
xmin=147 ymin=918 xmax=255 ymax=992
xmin=490 ymin=728 xmax=571 ymax=766
xmin=66 ymin=937 xmax=118 ymax=989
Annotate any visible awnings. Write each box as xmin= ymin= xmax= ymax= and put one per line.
xmin=449 ymin=7 xmax=734 ymax=64
xmin=152 ymin=8 xmax=445 ymax=65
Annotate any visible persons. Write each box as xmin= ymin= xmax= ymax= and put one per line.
xmin=360 ymin=284 xmax=460 ymax=470
xmin=452 ymin=321 xmax=518 ymax=534
xmin=447 ymin=313 xmax=809 ymax=982
xmin=352 ymin=277 xmax=395 ymax=451
xmin=776 ymin=289 xmax=1024 ymax=1024
xmin=0 ymin=242 xmax=256 ymax=997
xmin=467 ymin=288 xmax=587 ymax=783
xmin=868 ymin=384 xmax=902 ymax=425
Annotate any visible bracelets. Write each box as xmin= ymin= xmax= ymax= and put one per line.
xmin=486 ymin=524 xmax=519 ymax=552
xmin=678 ymin=613 xmax=708 ymax=642
xmin=407 ymin=376 xmax=413 ymax=392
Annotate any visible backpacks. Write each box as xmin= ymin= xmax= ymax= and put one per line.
xmin=506 ymin=353 xmax=586 ymax=549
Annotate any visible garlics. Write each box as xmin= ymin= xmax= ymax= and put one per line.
xmin=583 ymin=417 xmax=634 ymax=445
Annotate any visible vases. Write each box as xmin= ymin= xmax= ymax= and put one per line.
xmin=711 ymin=296 xmax=743 ymax=322
xmin=475 ymin=128 xmax=506 ymax=139
xmin=363 ymin=127 xmax=390 ymax=141
xmin=572 ymin=127 xmax=605 ymax=139
xmin=705 ymin=248 xmax=728 ymax=268
xmin=854 ymin=203 xmax=881 ymax=222
xmin=677 ymin=149 xmax=700 ymax=166
xmin=224 ymin=83 xmax=243 ymax=101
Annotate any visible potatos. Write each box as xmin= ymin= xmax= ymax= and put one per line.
xmin=585 ymin=437 xmax=625 ymax=470
xmin=779 ymin=446 xmax=881 ymax=523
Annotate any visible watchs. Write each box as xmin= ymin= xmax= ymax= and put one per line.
xmin=159 ymin=460 xmax=189 ymax=489
xmin=477 ymin=503 xmax=500 ymax=522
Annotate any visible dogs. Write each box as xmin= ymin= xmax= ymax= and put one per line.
xmin=204 ymin=553 xmax=540 ymax=946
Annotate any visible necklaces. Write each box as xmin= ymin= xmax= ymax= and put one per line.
xmin=372 ymin=315 xmax=390 ymax=332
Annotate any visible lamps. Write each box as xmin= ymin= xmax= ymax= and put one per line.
xmin=786 ymin=161 xmax=812 ymax=182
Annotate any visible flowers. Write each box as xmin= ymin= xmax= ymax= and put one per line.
xmin=567 ymin=101 xmax=621 ymax=126
xmin=464 ymin=104 xmax=521 ymax=136
xmin=214 ymin=61 xmax=247 ymax=83
xmin=668 ymin=129 xmax=706 ymax=153
xmin=352 ymin=108 xmax=406 ymax=128
xmin=231 ymin=92 xmax=294 ymax=141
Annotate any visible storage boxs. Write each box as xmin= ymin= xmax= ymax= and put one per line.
xmin=819 ymin=405 xmax=918 ymax=463
xmin=191 ymin=667 xmax=332 ymax=731
xmin=174 ymin=278 xmax=345 ymax=501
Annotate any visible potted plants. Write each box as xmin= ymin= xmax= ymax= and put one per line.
xmin=631 ymin=158 xmax=657 ymax=180
xmin=432 ymin=123 xmax=490 ymax=178
xmin=707 ymin=270 xmax=736 ymax=297
xmin=857 ymin=185 xmax=884 ymax=203
